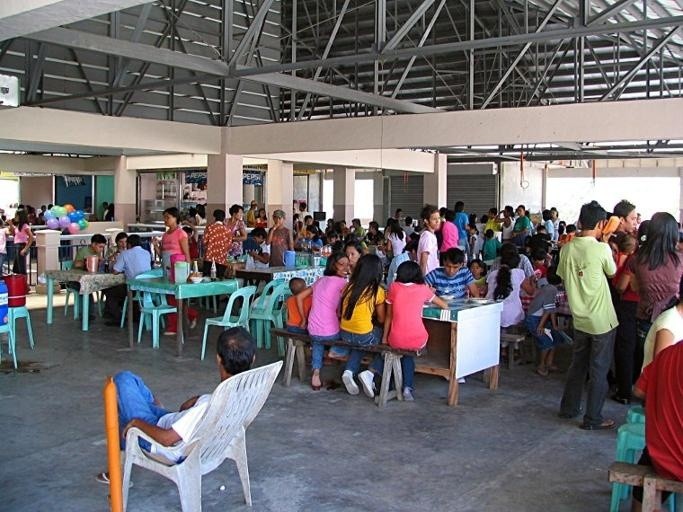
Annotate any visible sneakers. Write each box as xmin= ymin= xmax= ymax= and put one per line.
xmin=612 ymin=395 xmax=630 ymax=405
xmin=403 ymin=387 xmax=414 ymax=401
xmin=341 ymin=370 xmax=359 ymax=395
xmin=358 ymin=370 xmax=375 ymax=399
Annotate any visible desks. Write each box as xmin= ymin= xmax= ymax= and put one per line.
xmin=127 ymin=276 xmax=241 ymax=359
xmin=205 ymin=256 xmax=327 ymax=292
xmin=415 ymin=299 xmax=510 ymax=406
xmin=45 ymin=267 xmax=126 ymax=332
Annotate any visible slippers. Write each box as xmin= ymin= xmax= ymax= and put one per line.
xmin=585 ymin=419 xmax=616 ymax=430
xmin=532 ymin=367 xmax=549 ymax=377
xmin=94 ymin=473 xmax=134 ymax=488
xmin=548 ymin=361 xmax=560 ymax=372
xmin=557 ymin=406 xmax=583 ymax=418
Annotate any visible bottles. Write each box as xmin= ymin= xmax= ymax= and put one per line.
xmin=211 ymin=257 xmax=216 ymax=281
xmin=192 ymin=260 xmax=199 ymax=273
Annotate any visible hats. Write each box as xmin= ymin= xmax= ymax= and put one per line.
xmin=251 ymin=201 xmax=257 ymax=206
xmin=274 ymin=210 xmax=286 ymax=218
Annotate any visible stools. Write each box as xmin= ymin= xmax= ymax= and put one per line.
xmin=610 ymin=403 xmax=683 ymax=510
xmin=484 ymin=332 xmax=525 ymax=383
xmin=0 ymin=304 xmax=35 ymax=371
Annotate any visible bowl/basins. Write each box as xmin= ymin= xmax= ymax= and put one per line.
xmin=190 ymin=277 xmax=204 ymax=284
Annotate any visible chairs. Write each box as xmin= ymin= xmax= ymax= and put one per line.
xmin=120 ymin=268 xmax=184 ymax=349
xmin=60 ymin=260 xmax=102 ymax=320
xmin=117 ymin=360 xmax=286 ymax=512
xmin=197 ymin=273 xmax=292 ymax=361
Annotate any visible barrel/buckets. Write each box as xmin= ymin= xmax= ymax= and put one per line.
xmin=2 ymin=274 xmax=30 ymax=307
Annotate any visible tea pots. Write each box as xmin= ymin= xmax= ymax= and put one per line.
xmin=85 ymin=255 xmax=98 ymax=274
xmin=174 ymin=261 xmax=191 ymax=283
xmin=282 ymin=250 xmax=295 ymax=270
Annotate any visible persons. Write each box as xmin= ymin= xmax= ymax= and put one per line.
xmin=0 ymin=195 xmax=683 ymax=406
xmin=95 ymin=323 xmax=258 ymax=485
xmin=629 ymin=337 xmax=682 ymax=512
xmin=554 ymin=198 xmax=622 ymax=429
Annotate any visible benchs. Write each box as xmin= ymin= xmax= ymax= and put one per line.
xmin=270 ymin=325 xmax=428 ymax=408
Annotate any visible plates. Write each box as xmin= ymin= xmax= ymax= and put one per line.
xmin=469 ymin=298 xmax=490 ymax=305
xmin=440 ymin=294 xmax=454 ymax=300
